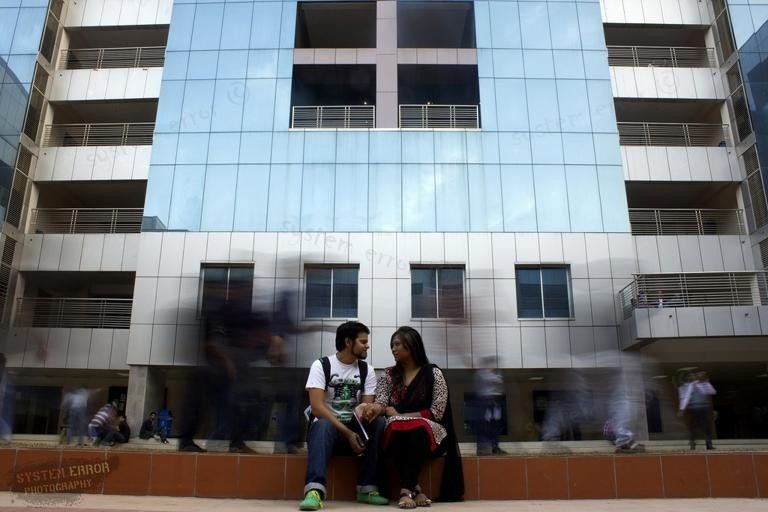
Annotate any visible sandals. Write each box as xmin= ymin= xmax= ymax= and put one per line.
xmin=413 ymin=489 xmax=432 ymax=506
xmin=398 ymin=493 xmax=417 ymax=508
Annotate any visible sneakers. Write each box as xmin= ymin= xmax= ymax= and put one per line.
xmin=178 ymin=442 xmax=207 ymax=452
xmin=228 ymin=442 xmax=257 ymax=453
xmin=356 ymin=491 xmax=389 ymax=504
xmin=299 ymin=490 xmax=322 ymax=510
xmin=475 ymin=440 xmax=720 ymax=455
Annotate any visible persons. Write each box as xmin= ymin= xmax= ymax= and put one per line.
xmin=2 ymin=275 xmax=717 ymax=457
xmin=359 ymin=323 xmax=449 ymax=512
xmin=297 ymin=320 xmax=393 ymax=511
